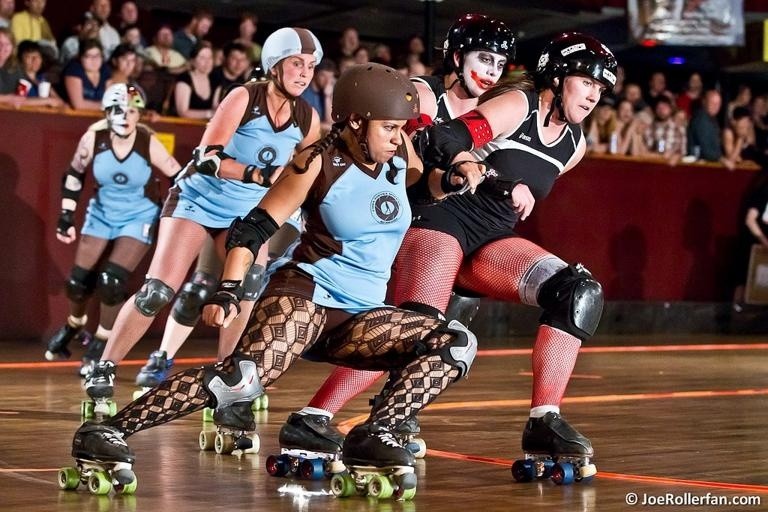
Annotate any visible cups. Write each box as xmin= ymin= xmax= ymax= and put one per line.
xmin=38 ymin=81 xmax=51 ymax=98
xmin=16 ymin=79 xmax=32 ymax=97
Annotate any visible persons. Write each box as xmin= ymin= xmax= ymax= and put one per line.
xmin=0 ymin=2 xmax=768 ymax=172
xmin=49 ymin=79 xmax=189 ymax=362
xmin=210 ymin=16 xmax=515 ymax=435
xmin=723 ymin=176 xmax=768 ymax=325
xmin=71 ymin=62 xmax=486 ymax=466
xmin=279 ymin=30 xmax=621 ymax=457
xmin=85 ymin=26 xmax=325 ymax=397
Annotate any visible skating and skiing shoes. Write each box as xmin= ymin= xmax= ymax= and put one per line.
xmin=367 ymin=395 xmax=426 ymax=458
xmin=267 ymin=412 xmax=346 ymax=481
xmin=78 ymin=359 xmax=118 ymax=421
xmin=58 ymin=421 xmax=137 ymax=495
xmin=199 ymin=400 xmax=260 ymax=455
xmin=511 ymin=411 xmax=597 ymax=486
xmin=330 ymin=423 xmax=417 ymax=501
xmin=47 ymin=317 xmax=91 ymax=359
xmin=132 ymin=350 xmax=174 ymax=400
xmin=203 ymin=390 xmax=269 ymax=423
xmin=83 ymin=335 xmax=107 ymax=362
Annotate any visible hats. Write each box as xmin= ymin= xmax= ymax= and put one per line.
xmin=79 ymin=10 xmax=101 ymax=29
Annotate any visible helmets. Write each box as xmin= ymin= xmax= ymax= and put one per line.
xmin=261 ymin=27 xmax=325 ymax=75
xmin=330 ymin=62 xmax=421 ymax=123
xmin=533 ymin=31 xmax=619 ymax=90
xmin=100 ymin=82 xmax=148 ymax=111
xmin=442 ymin=11 xmax=517 ymax=62
